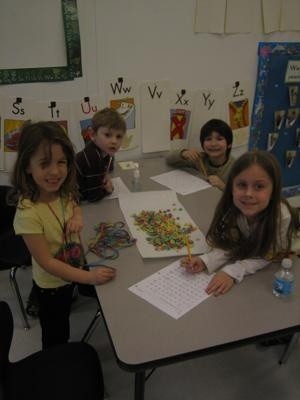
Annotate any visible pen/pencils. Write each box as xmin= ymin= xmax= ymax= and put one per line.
xmin=101 ymin=156 xmax=112 ymax=183
xmin=199 ymin=158 xmax=208 ymax=177
xmin=185 ymin=234 xmax=193 ymax=270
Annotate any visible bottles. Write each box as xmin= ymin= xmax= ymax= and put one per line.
xmin=272 ymin=258 xmax=294 ymax=298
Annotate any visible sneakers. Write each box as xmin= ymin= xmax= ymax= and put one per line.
xmin=27 ymin=300 xmax=40 ymax=316
xmin=261 ymin=335 xmax=292 ymax=346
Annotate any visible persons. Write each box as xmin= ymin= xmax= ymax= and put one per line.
xmin=167 ymin=118 xmax=239 ymax=189
xmin=182 ymin=147 xmax=300 ymax=296
xmin=74 ymin=105 xmax=126 ymax=204
xmin=13 ymin=121 xmax=115 ymax=365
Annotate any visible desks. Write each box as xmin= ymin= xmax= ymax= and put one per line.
xmin=71 ymin=157 xmax=300 ymax=400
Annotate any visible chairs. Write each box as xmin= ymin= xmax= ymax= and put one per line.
xmin=0 ymin=185 xmax=31 ymax=330
xmin=1 ymin=301 xmax=105 ymax=400
